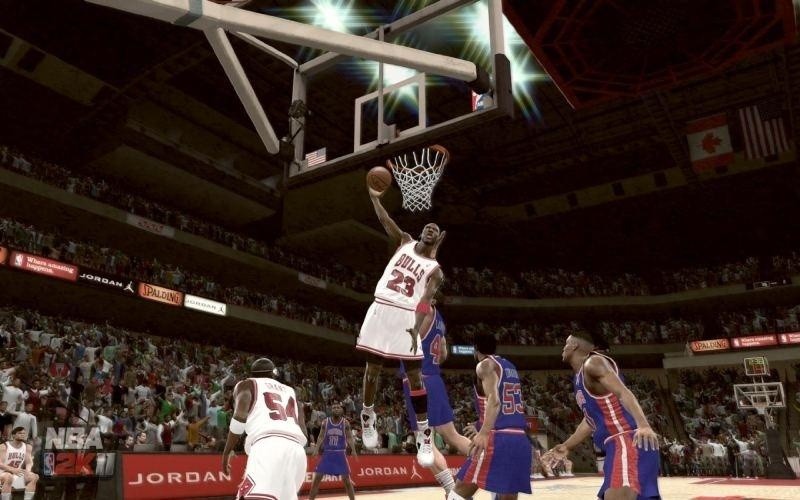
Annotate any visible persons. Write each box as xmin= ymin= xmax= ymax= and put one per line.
xmin=1 ymin=143 xmax=800 ymax=499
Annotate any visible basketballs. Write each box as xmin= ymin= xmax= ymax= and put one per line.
xmin=366 ymin=166 xmax=391 ymax=191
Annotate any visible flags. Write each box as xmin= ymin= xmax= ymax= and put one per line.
xmin=686 ymin=110 xmax=736 ymax=179
xmin=737 ymin=94 xmax=789 ymax=162
